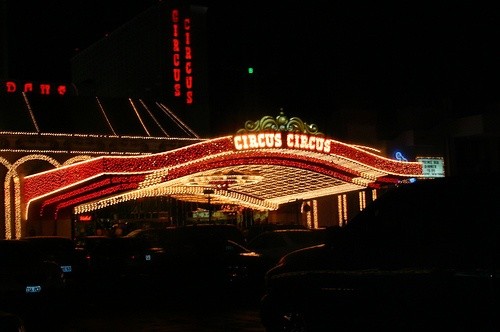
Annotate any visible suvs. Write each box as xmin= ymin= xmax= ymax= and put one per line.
xmin=259 ymin=177 xmax=500 ymax=332
xmin=0 ymin=221 xmax=328 ymax=332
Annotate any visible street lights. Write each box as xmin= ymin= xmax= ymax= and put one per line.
xmin=204 ymin=190 xmax=213 ymax=224
xmin=296 ymin=198 xmax=310 ymax=224
